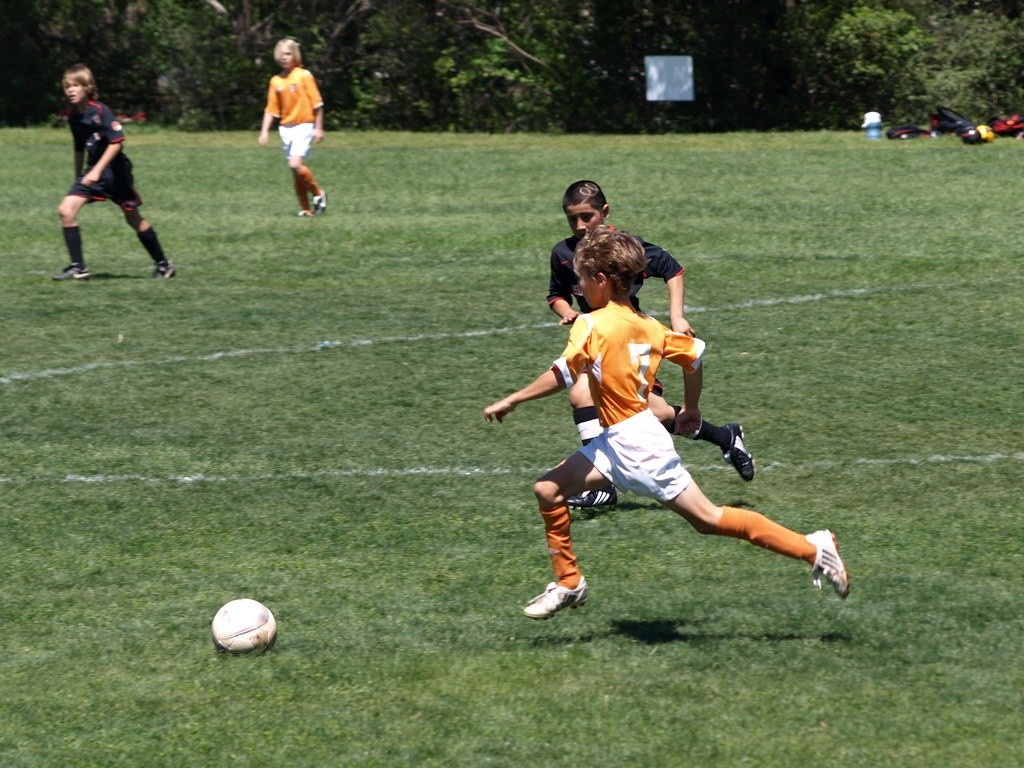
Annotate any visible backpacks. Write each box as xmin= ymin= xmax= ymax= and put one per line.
xmin=985 ymin=114 xmax=1024 ymax=132
xmin=887 ymin=124 xmax=930 ymax=138
xmin=927 ymin=106 xmax=977 ymax=136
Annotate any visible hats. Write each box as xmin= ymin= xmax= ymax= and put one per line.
xmin=861 ymin=111 xmax=881 ymax=128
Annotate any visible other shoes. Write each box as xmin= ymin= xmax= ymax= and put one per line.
xmin=298 ymin=209 xmax=312 ymax=217
xmin=312 ymin=190 xmax=327 ymax=216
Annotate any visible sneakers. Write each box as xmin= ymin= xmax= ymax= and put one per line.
xmin=566 ymin=486 xmax=618 ymax=506
xmin=523 ymin=575 xmax=588 ymax=620
xmin=806 ymin=528 xmax=851 ymax=600
xmin=155 ymin=259 xmax=175 ymax=278
xmin=52 ymin=261 xmax=90 ymax=280
xmin=720 ymin=422 xmax=757 ymax=482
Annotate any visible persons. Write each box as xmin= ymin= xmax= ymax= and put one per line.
xmin=52 ymin=64 xmax=177 ymax=282
xmin=259 ymin=39 xmax=327 ymax=219
xmin=546 ymin=181 xmax=754 ymax=509
xmin=482 ymin=224 xmax=850 ymax=620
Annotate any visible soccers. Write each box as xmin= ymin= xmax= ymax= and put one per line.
xmin=211 ymin=599 xmax=277 ymax=657
